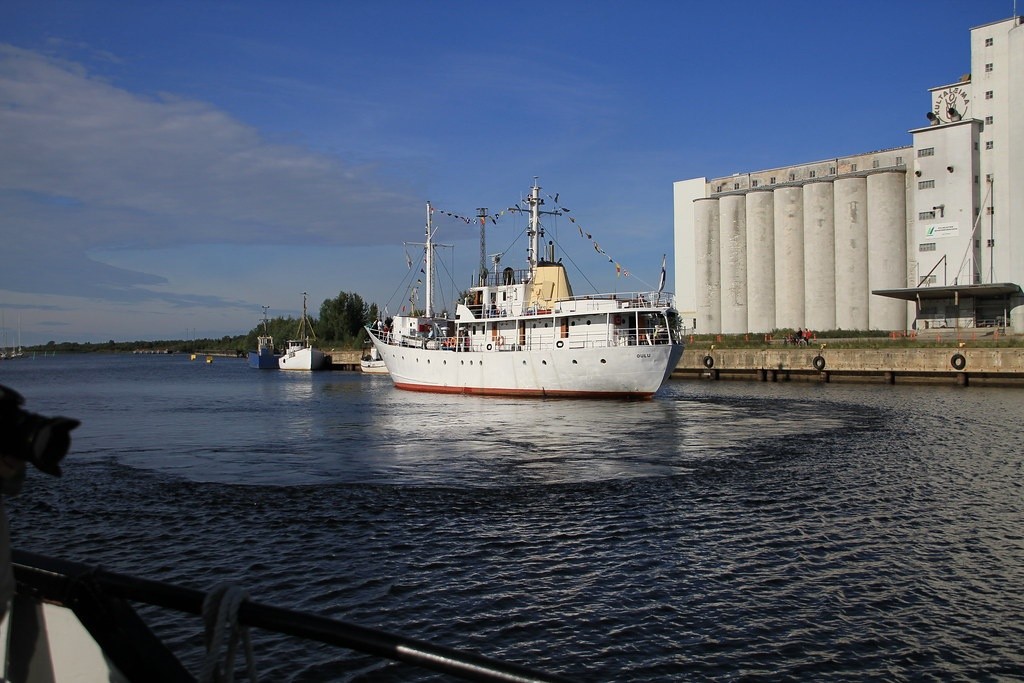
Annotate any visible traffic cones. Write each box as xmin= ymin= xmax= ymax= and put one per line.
xmin=892 ymin=332 xmax=896 ymax=340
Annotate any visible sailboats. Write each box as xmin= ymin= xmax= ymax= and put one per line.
xmin=278 ymin=292 xmax=324 ymax=370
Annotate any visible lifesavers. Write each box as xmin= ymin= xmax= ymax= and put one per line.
xmin=614 ymin=315 xmax=621 ymax=325
xmin=951 ymin=354 xmax=966 ymax=369
xmin=498 ymin=336 xmax=504 ymax=346
xmin=813 ymin=356 xmax=825 ymax=369
xmin=450 ymin=339 xmax=456 ymax=346
xmin=703 ymin=356 xmax=713 ymax=368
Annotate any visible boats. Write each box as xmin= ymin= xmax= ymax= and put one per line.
xmin=248 ymin=305 xmax=282 ymax=369
xmin=363 ymin=176 xmax=686 ymax=398
xmin=360 ymin=348 xmax=389 ymax=373
xmin=401 ymin=335 xmax=443 ymax=350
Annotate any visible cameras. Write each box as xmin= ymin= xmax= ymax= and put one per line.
xmin=0 ymin=383 xmax=82 ymax=494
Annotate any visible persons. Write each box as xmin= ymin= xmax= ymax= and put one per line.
xmin=796 ymin=327 xmax=811 ymax=346
xmin=460 ymin=331 xmax=465 ymax=352
xmin=492 ymin=301 xmax=496 ymax=315
xmin=377 ymin=316 xmax=393 ymax=340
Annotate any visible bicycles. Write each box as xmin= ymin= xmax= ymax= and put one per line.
xmin=783 ymin=335 xmax=807 ymax=346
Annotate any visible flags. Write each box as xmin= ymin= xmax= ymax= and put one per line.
xmin=547 ymin=193 xmax=628 ymax=278
xmin=430 ymin=199 xmax=526 ymax=225
xmin=658 ymin=259 xmax=666 ymax=291
xmin=402 ymin=250 xmax=426 ymax=312
